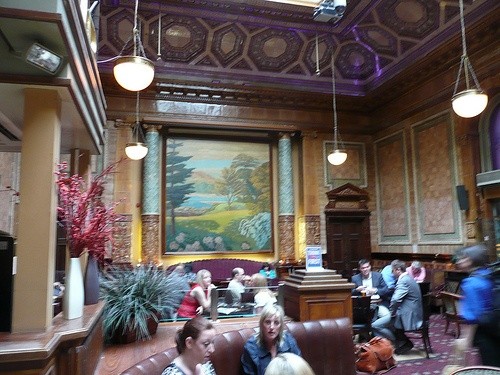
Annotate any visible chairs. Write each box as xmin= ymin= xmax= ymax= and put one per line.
xmin=352 ymin=306 xmax=379 ymax=343
xmin=403 ymin=281 xmax=432 ymax=359
xmin=440 ymin=271 xmax=469 ymax=339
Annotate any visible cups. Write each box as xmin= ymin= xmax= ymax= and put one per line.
xmin=361 ymin=291 xmax=366 ymax=296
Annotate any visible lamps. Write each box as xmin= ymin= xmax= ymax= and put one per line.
xmin=125 ymin=92 xmax=148 ymax=160
xmin=9 ymin=42 xmax=65 ymax=75
xmin=327 ymin=49 xmax=347 ymax=166
xmin=451 ymin=0 xmax=488 ymax=118
xmin=112 ymin=0 xmax=155 ymax=92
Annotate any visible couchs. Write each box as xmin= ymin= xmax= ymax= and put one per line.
xmin=166 ymin=258 xmax=263 ymax=280
xmin=120 ymin=317 xmax=356 ymax=375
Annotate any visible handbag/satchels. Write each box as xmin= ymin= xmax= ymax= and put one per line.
xmin=352 ymin=335 xmax=396 ymax=375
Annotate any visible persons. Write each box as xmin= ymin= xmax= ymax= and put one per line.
xmin=351 ymin=243 xmax=500 ymax=368
xmin=162 ymin=262 xmax=315 ymax=375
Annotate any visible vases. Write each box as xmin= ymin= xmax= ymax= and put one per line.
xmin=84 ymin=250 xmax=99 ymax=305
xmin=63 ymin=257 xmax=84 ymax=319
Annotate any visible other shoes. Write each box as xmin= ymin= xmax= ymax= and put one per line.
xmin=394 ymin=342 xmax=414 ymax=354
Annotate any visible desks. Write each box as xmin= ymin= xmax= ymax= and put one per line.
xmin=277 ymin=265 xmax=306 ymax=273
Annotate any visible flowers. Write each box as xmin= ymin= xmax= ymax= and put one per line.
xmin=53 ymin=156 xmax=131 ymax=256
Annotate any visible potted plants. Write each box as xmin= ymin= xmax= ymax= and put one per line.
xmin=97 ymin=261 xmax=197 ymax=345
